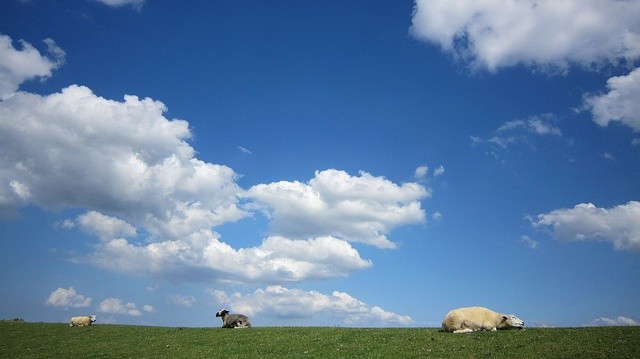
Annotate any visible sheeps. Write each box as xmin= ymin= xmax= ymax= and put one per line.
xmin=70 ymin=315 xmax=96 ymax=327
xmin=216 ymin=310 xmax=251 ymax=328
xmin=443 ymin=306 xmax=525 ymax=334
